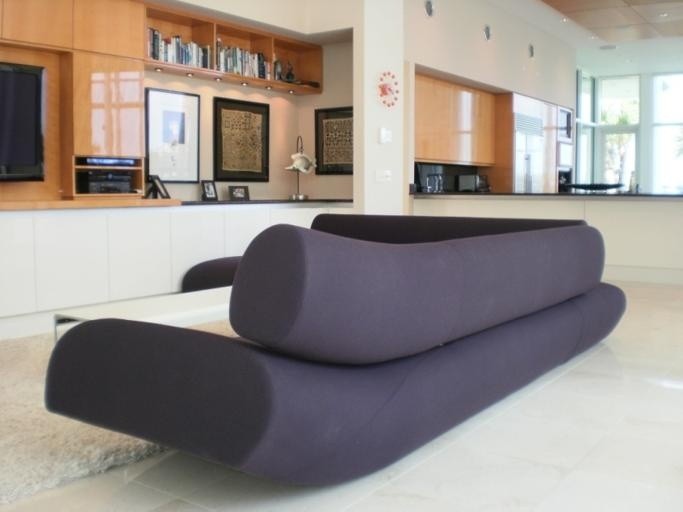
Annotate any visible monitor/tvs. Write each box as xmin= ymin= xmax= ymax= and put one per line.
xmin=0 ymin=62 xmax=46 ymax=184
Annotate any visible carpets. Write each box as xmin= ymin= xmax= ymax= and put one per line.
xmin=0 ymin=334 xmax=168 ymax=506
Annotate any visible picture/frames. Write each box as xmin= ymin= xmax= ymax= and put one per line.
xmin=212 ymin=97 xmax=269 ymax=183
xmin=315 ymin=106 xmax=353 ymax=174
xmin=201 ymin=180 xmax=218 ymax=201
xmin=228 ymin=186 xmax=250 ymax=201
xmin=145 ymin=87 xmax=200 ymax=183
xmin=150 ymin=176 xmax=171 ymax=199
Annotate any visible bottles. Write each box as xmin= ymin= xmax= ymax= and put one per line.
xmin=427 ymin=174 xmax=443 ymax=192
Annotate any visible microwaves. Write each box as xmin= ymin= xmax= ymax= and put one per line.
xmin=458 ymin=175 xmax=489 ymax=192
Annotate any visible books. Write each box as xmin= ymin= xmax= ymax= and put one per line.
xmin=144 ymin=25 xmax=271 ymax=82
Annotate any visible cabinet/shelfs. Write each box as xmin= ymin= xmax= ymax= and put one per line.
xmin=414 ymin=73 xmax=576 ymax=194
xmin=59 ymin=52 xmax=144 ymax=202
xmin=1 ymin=0 xmax=324 ymax=95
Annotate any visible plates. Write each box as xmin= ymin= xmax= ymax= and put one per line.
xmin=565 ymin=183 xmax=625 ymax=191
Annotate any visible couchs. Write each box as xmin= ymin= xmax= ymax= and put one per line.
xmin=45 ymin=214 xmax=627 ymax=490
